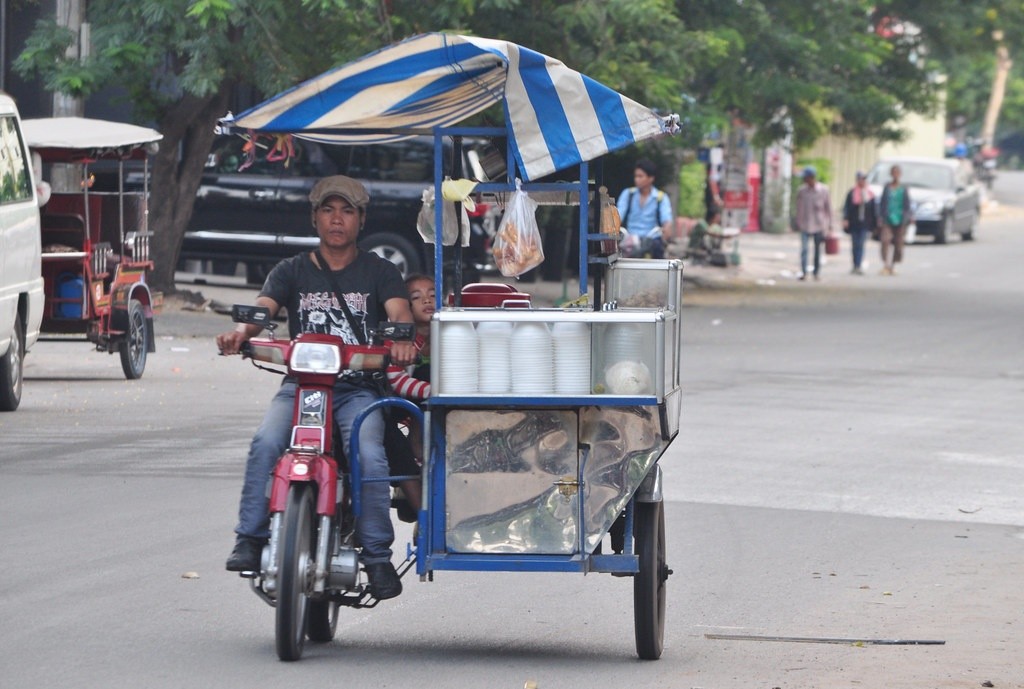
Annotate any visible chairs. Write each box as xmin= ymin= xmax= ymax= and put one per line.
xmin=41 ymin=214 xmax=86 ymax=267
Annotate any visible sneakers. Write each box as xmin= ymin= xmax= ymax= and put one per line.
xmin=366 ymin=560 xmax=402 ymax=600
xmin=225 ymin=535 xmax=268 ymax=572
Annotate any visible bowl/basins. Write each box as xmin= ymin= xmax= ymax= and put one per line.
xmin=439 ymin=321 xmax=592 ymax=395
xmin=602 ymin=323 xmax=644 ymax=394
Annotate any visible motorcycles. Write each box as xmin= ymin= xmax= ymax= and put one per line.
xmin=967 ymin=139 xmax=999 ymax=189
xmin=17 ymin=116 xmax=160 ymax=382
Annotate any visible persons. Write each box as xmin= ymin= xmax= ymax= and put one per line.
xmin=955 ymin=144 xmax=971 ymax=170
xmin=216 ymin=177 xmax=417 ymax=599
xmin=878 ymin=166 xmax=912 ymax=274
xmin=797 ymin=167 xmax=833 ymax=281
xmin=845 ymin=173 xmax=878 ymax=276
xmin=617 ymin=161 xmax=672 ymax=259
xmin=704 ymin=143 xmax=727 ymax=247
xmin=384 ymin=273 xmax=446 ymax=547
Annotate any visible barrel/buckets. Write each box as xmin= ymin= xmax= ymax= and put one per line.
xmin=823 ymin=235 xmax=838 ymax=253
xmin=55 ymin=269 xmax=83 ymax=320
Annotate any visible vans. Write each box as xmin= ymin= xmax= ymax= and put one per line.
xmin=0 ymin=94 xmax=46 ymax=410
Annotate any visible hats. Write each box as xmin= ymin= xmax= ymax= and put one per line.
xmin=799 ymin=168 xmax=815 ymax=177
xmin=307 ymin=174 xmax=370 ymax=212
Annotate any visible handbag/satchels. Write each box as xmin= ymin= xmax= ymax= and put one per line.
xmin=825 ymin=238 xmax=837 ymax=254
xmin=494 ymin=179 xmax=545 ymax=277
xmin=416 ymin=187 xmax=470 ymax=247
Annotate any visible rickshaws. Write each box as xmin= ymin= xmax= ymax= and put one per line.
xmin=208 ymin=37 xmax=681 ymax=664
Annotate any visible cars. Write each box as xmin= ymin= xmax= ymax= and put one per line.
xmin=119 ymin=131 xmax=514 ymax=295
xmin=842 ymin=158 xmax=982 ymax=246
